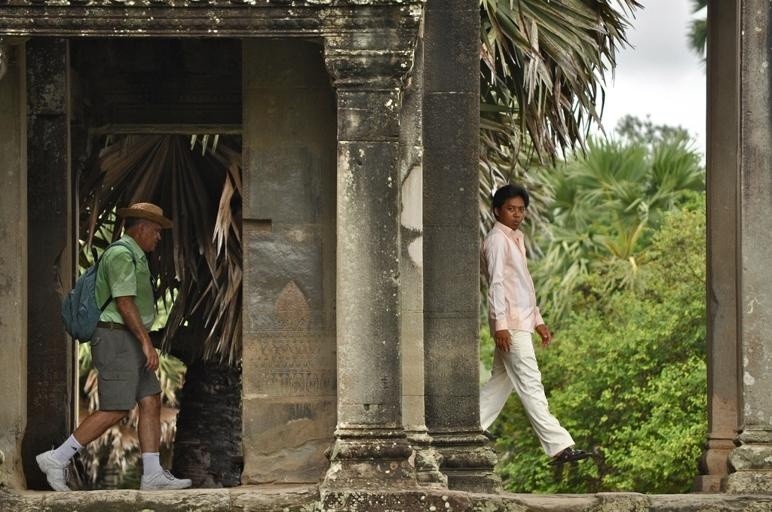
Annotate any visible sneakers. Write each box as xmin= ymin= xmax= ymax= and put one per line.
xmin=139 ymin=470 xmax=192 ymax=490
xmin=35 ymin=449 xmax=71 ymax=492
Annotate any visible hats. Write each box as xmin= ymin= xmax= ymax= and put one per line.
xmin=117 ymin=202 xmax=175 ymax=230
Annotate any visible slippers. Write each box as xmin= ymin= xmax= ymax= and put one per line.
xmin=548 ymin=447 xmax=594 ymax=467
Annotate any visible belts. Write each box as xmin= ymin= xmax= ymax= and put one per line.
xmin=96 ymin=320 xmax=131 ymax=332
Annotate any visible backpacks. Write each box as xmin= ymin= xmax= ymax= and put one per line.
xmin=60 ymin=239 xmax=137 ymax=344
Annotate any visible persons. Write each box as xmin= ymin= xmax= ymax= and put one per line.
xmin=480 ymin=183 xmax=593 ymax=465
xmin=34 ymin=202 xmax=192 ymax=490
